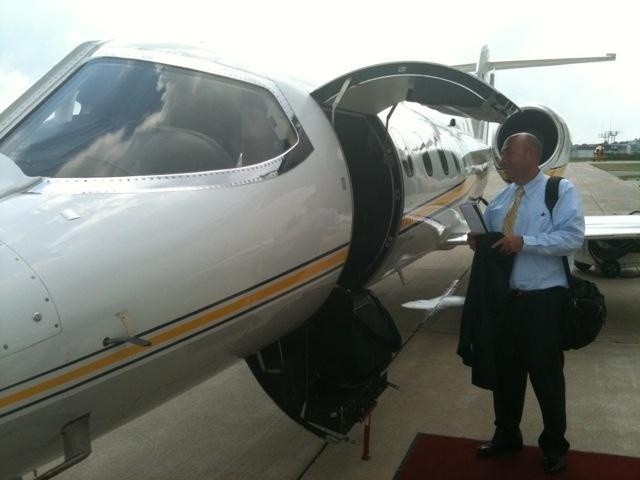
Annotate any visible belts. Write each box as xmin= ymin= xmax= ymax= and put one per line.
xmin=510 ymin=288 xmax=553 ymax=297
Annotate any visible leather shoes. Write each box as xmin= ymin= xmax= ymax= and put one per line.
xmin=476 ymin=440 xmax=521 ymax=456
xmin=542 ymin=451 xmax=565 ymax=475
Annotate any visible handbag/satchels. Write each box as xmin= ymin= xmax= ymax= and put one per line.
xmin=558 ymin=275 xmax=608 ymax=351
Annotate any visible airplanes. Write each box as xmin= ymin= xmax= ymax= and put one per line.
xmin=0 ymin=38 xmax=640 ymax=478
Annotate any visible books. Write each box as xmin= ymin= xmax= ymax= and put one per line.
xmin=460 ymin=201 xmax=490 ymax=236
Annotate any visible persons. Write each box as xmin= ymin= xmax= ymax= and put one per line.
xmin=465 ymin=132 xmax=585 ymax=474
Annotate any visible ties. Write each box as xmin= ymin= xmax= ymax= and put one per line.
xmin=502 ymin=187 xmax=524 ymax=238
